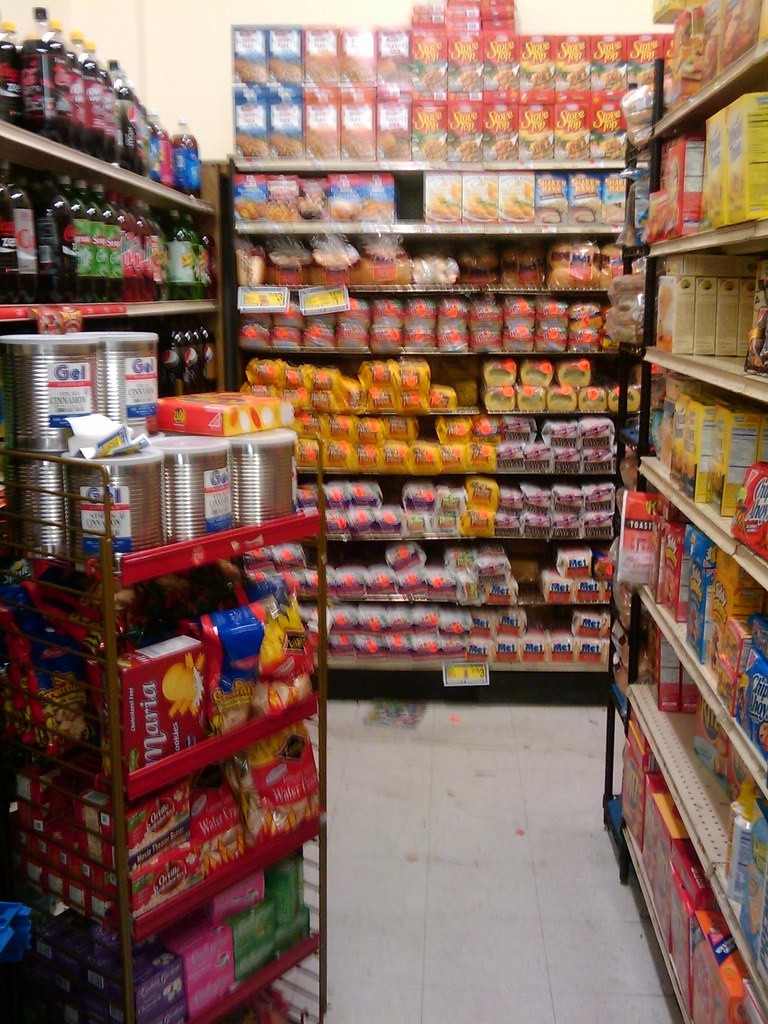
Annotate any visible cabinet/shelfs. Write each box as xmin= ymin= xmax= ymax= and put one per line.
xmin=602 ymin=48 xmax=768 ymax=1024
xmin=224 ymin=159 xmax=623 ymax=678
xmin=0 ymin=117 xmax=224 ymax=512
xmin=0 ymin=432 xmax=329 ymax=1024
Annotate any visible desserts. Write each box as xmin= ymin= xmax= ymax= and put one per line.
xmin=162 ymin=664 xmax=197 ymax=700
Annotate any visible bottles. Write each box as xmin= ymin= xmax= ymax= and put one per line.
xmin=0 ymin=8 xmax=216 ymax=305
xmin=100 ymin=314 xmax=216 ymax=399
xmin=727 ymin=798 xmax=755 ymax=904
xmin=725 ymin=779 xmax=755 ymax=879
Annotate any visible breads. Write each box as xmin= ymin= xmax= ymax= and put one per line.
xmin=572 ymin=207 xmax=595 ymax=222
xmin=537 ymin=208 xmax=561 ymax=222
xmin=239 ymin=234 xmax=639 ymax=665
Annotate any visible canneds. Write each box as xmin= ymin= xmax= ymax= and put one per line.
xmin=1 ymin=331 xmax=296 ymax=572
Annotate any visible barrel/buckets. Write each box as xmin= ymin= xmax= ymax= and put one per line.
xmin=67 ymin=332 xmax=158 ymax=439
xmin=60 ymin=452 xmax=165 ymax=572
xmin=229 ymin=427 xmax=298 ymax=526
xmin=0 ymin=334 xmax=99 ymax=451
xmin=4 ymin=453 xmax=72 ymax=559
xmin=150 ymin=437 xmax=233 ymax=545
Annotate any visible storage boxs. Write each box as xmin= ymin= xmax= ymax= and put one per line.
xmin=152 ymin=386 xmax=294 ymax=431
xmin=618 ymin=371 xmax=768 ymax=1023
xmin=12 ymin=757 xmax=311 ymax=1024
xmin=114 ymin=634 xmax=206 ymax=774
xmin=653 ymin=252 xmax=762 ymax=357
xmin=226 ymin=0 xmax=767 ymax=249
